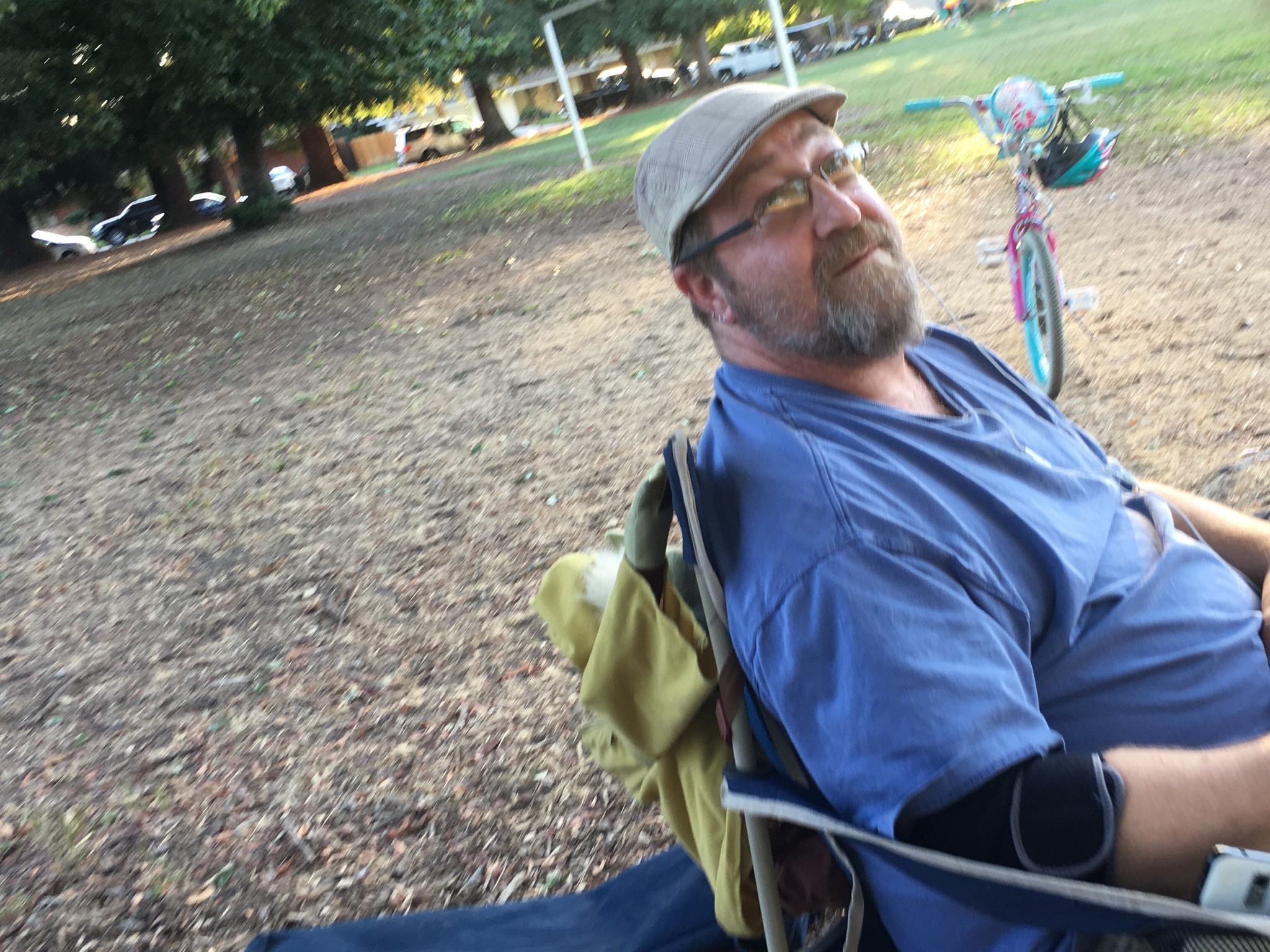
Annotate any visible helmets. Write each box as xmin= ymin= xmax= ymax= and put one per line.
xmin=1037 ymin=126 xmax=1121 ymax=189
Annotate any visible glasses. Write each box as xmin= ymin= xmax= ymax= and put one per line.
xmin=675 ymin=141 xmax=874 ymax=267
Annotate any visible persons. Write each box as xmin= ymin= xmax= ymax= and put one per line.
xmin=621 ymin=71 xmax=1270 ymax=952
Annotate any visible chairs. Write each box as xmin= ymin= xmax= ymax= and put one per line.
xmin=653 ymin=429 xmax=1270 ymax=952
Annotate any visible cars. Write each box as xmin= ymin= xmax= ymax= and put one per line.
xmin=32 ymin=16 xmax=840 ymax=259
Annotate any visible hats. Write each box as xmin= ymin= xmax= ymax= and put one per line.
xmin=634 ymin=82 xmax=845 ymax=265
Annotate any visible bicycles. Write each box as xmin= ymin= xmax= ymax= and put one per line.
xmin=906 ymin=71 xmax=1125 ymax=400
